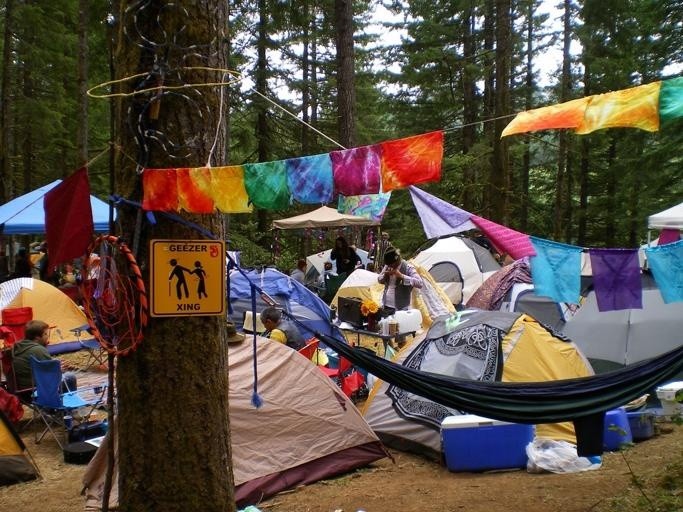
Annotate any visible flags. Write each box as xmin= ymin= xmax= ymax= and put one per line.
xmin=500 ymin=76 xmax=683 ymax=138
xmin=140 ymin=131 xmax=445 ymax=216
xmin=409 ymin=184 xmax=478 ymax=238
xmin=469 ymin=213 xmax=537 ymax=260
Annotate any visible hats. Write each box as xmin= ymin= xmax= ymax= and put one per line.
xmin=384 ymin=247 xmax=399 ymax=264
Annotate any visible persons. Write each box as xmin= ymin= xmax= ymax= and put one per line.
xmin=5 ymin=319 xmax=78 ymax=403
xmin=368 ymin=232 xmax=395 ymax=272
xmin=290 ymin=238 xmax=425 ymax=312
xmin=56 ymin=259 xmax=83 ymax=287
xmin=15 ymin=240 xmax=50 ymax=281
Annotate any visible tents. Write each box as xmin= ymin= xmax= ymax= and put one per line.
xmin=303 ymin=240 xmax=683 ymax=469
xmin=81 ymin=264 xmax=397 ymax=511
xmin=0 ymin=274 xmax=101 ymax=354
